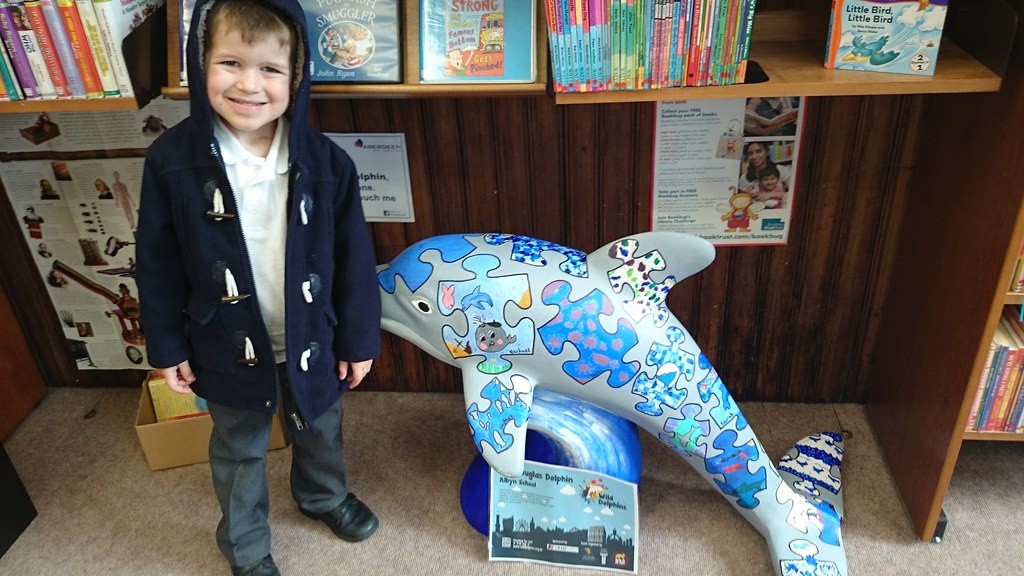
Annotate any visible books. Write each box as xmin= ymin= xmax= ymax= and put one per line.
xmin=297 ymin=0 xmax=402 ymax=83
xmin=418 ymin=0 xmax=536 ymax=85
xmin=178 ymin=0 xmax=197 ymax=86
xmin=746 ymin=108 xmax=799 ymax=128
xmin=148 ymin=378 xmax=209 ymax=422
xmin=965 ymin=241 xmax=1024 ymax=434
xmin=824 ymin=0 xmax=949 ymax=76
xmin=0 ymin=0 xmax=164 ymax=101
xmin=544 ymin=0 xmax=758 ymax=93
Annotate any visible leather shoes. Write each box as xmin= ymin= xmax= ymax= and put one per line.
xmin=298 ymin=490 xmax=380 ymax=542
xmin=230 ymin=552 xmax=280 ymax=576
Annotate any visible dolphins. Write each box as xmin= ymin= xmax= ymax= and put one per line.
xmin=374 ymin=231 xmax=850 ymax=576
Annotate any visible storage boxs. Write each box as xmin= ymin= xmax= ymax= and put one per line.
xmin=823 ymin=0 xmax=948 ymax=76
xmin=134 ymin=370 xmax=287 ymax=471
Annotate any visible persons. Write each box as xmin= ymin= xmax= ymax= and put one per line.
xmin=22 ymin=206 xmax=44 ymax=239
xmin=134 ymin=0 xmax=381 ymax=576
xmin=738 ymin=141 xmax=792 ymax=210
xmin=95 ymin=179 xmax=114 ymax=199
xmin=40 ymin=179 xmax=60 ymax=200
xmin=47 ymin=271 xmax=67 ymax=288
xmin=744 ymin=97 xmax=798 ymax=136
xmin=39 ymin=244 xmax=52 ymax=259
xmin=128 ymin=347 xmax=143 ymax=365
xmin=76 ymin=323 xmax=90 ymax=337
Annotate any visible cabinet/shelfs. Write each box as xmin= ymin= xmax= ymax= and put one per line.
xmin=867 ymin=0 xmax=1024 ymax=543
